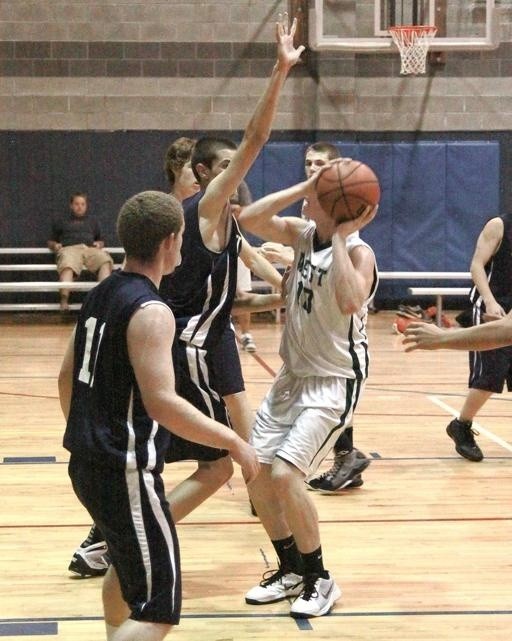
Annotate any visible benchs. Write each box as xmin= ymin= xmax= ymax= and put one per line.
xmin=0 ymin=248 xmax=127 ymax=313
xmin=245 ymin=263 xmax=474 ymax=327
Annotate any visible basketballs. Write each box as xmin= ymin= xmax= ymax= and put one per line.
xmin=317 ymin=161 xmax=380 ymax=221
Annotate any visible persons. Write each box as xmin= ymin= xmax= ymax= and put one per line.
xmin=47 ymin=189 xmax=114 ymax=316
xmin=303 ymin=140 xmax=374 ymax=493
xmin=66 ymin=8 xmax=309 ymax=579
xmin=400 ymin=308 xmax=512 ymax=356
xmin=56 ymin=191 xmax=261 ymax=641
xmin=446 ymin=207 xmax=512 ymax=460
xmin=163 ymin=135 xmax=283 ymax=519
xmin=229 ymin=176 xmax=256 ymax=352
xmin=237 ymin=155 xmax=380 ymax=623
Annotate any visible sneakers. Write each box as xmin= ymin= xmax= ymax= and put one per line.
xmin=245 ymin=561 xmax=302 ymax=605
xmin=289 ymin=570 xmax=343 ymax=617
xmin=447 ymin=417 xmax=483 ymax=462
xmin=396 ymin=304 xmax=432 ymax=322
xmin=309 ymin=447 xmax=371 ymax=493
xmin=68 ymin=539 xmax=112 ymax=577
xmin=240 ymin=332 xmax=257 ymax=352
